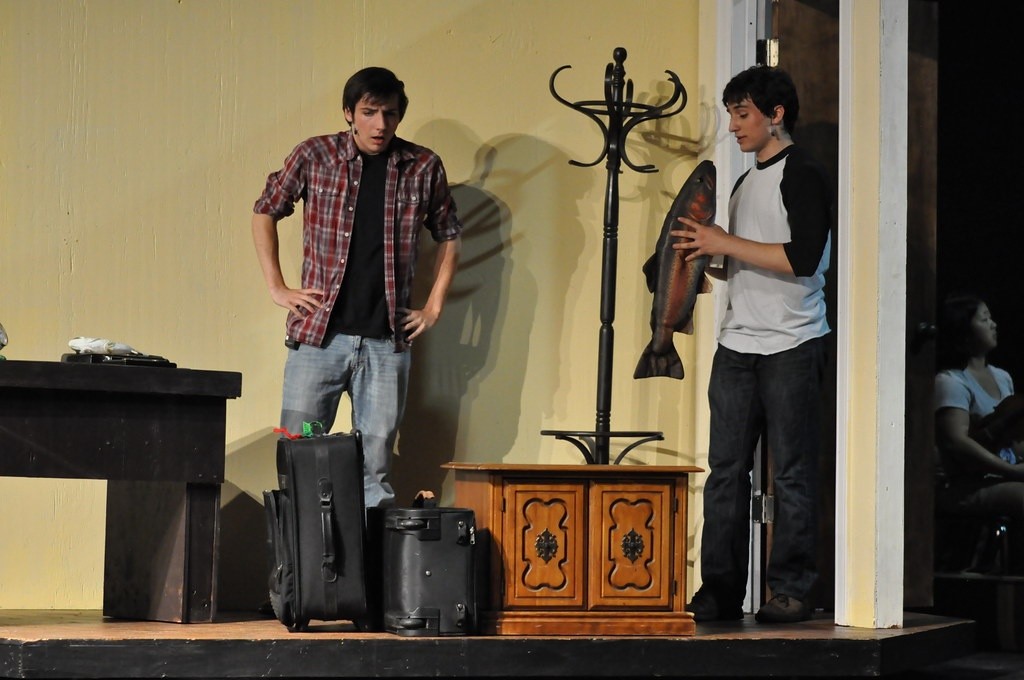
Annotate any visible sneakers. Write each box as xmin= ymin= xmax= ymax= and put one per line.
xmin=685 ymin=585 xmax=745 ymax=621
xmin=755 ymin=593 xmax=821 ymax=623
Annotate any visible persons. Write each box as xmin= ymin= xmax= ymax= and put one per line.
xmin=934 ymin=290 xmax=1024 ymax=518
xmin=251 ymin=66 xmax=463 ymax=507
xmin=670 ymin=64 xmax=832 ymax=622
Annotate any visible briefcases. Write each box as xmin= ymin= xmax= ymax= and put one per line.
xmin=262 ymin=420 xmax=370 ymax=633
xmin=383 ymin=490 xmax=478 ymax=637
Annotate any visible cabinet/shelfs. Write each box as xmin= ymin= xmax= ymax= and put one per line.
xmin=441 ymin=462 xmax=704 ymax=636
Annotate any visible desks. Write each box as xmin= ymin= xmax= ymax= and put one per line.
xmin=0 ymin=359 xmax=242 ymax=624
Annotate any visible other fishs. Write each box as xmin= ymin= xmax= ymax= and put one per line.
xmin=633 ymin=159 xmax=727 ymax=380
